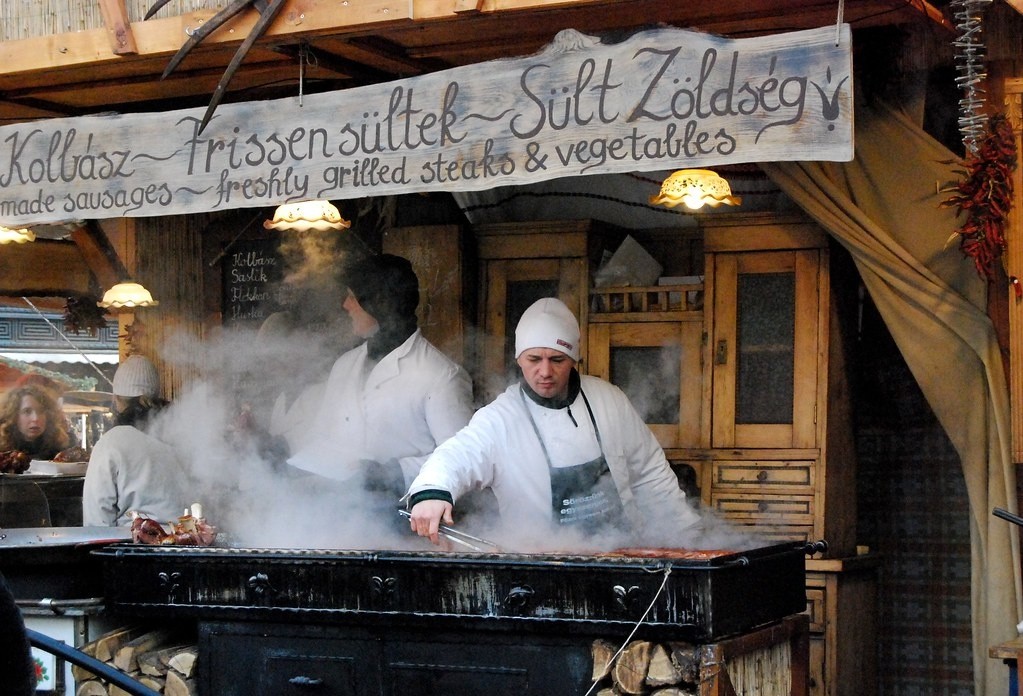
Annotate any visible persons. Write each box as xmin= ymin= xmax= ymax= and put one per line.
xmin=63 ymin=407 xmax=115 ymax=449
xmin=0 ymin=375 xmax=79 ymax=460
xmin=398 ymin=297 xmax=704 ymax=547
xmin=239 ymin=255 xmax=474 ymax=544
xmin=81 ymin=356 xmax=216 ymax=531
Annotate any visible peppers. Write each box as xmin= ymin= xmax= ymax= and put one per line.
xmin=929 ymin=113 xmax=1020 ymax=282
xmin=59 ymin=295 xmax=111 ymax=336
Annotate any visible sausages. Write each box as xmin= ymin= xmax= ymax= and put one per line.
xmin=612 ymin=546 xmax=736 ymax=559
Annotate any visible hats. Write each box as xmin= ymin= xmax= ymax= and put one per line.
xmin=345 ymin=253 xmax=421 ymax=332
xmin=514 ymin=296 xmax=582 ymax=365
xmin=112 ymin=354 xmax=160 ymax=398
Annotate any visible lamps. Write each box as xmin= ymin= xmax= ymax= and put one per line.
xmin=97 ymin=221 xmax=159 ymax=309
xmin=648 ymin=169 xmax=741 ymax=210
xmin=0 ymin=225 xmax=37 ymax=246
xmin=263 ymin=200 xmax=352 ymax=234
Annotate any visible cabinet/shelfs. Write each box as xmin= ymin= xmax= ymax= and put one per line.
xmin=474 ymin=209 xmax=891 ymax=696
